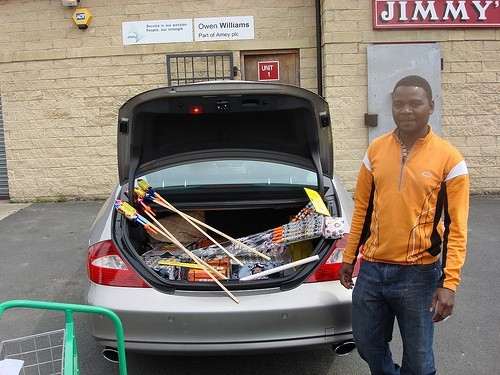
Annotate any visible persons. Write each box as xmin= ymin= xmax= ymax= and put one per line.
xmin=337 ymin=74 xmax=470 ymax=375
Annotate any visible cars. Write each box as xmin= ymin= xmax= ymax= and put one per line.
xmin=83 ymin=79 xmax=365 ymax=364
